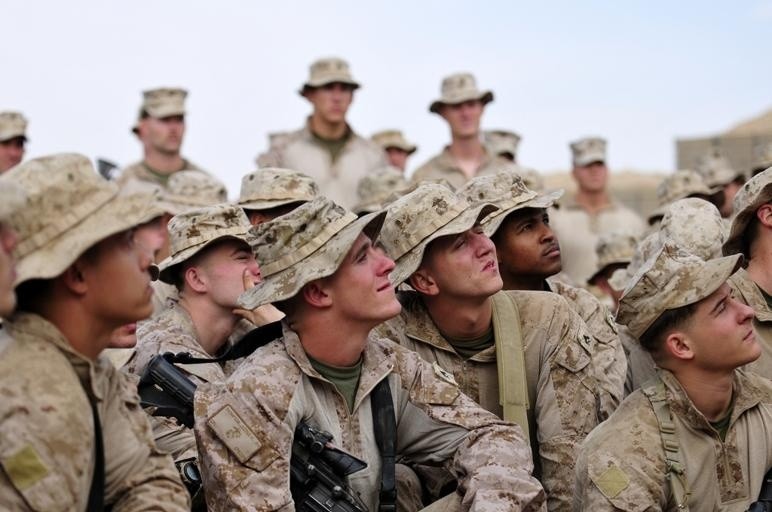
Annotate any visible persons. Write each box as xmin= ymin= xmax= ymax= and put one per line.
xmin=114 ymin=87 xmax=212 ymax=186
xmin=0 ymin=152 xmax=771 ymax=512
xmin=0 ymin=110 xmax=29 ymax=175
xmin=560 ymin=136 xmax=621 ymax=214
xmin=414 ymin=72 xmax=522 ymax=192
xmin=256 ymin=57 xmax=417 ymax=210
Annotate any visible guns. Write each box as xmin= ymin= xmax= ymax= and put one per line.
xmin=135 ymin=354 xmax=369 ymax=511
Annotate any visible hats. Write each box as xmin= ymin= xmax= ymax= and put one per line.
xmin=156 ymin=170 xmax=234 ymax=218
xmin=0 ymin=111 xmax=29 ymax=142
xmin=237 ymin=194 xmax=388 ymax=314
xmin=646 ymin=168 xmax=726 ymax=227
xmin=607 ymin=196 xmax=734 ymax=292
xmin=720 ymin=165 xmax=772 ymax=258
xmin=568 ymin=136 xmax=607 ymax=167
xmin=234 ymin=166 xmax=322 ymax=210
xmin=455 ymin=171 xmax=567 ymax=239
xmin=297 ymin=56 xmax=361 ymax=95
xmin=0 ymin=150 xmax=162 ymax=289
xmin=697 ymin=156 xmax=749 ymax=188
xmin=377 ymin=177 xmax=503 ymax=292
xmin=428 ymin=73 xmax=495 ymax=113
xmin=139 ymin=86 xmax=188 ymax=120
xmin=348 ymin=165 xmax=409 ymax=215
xmin=614 ymin=239 xmax=745 ymax=342
xmin=371 ymin=129 xmax=418 ymax=155
xmin=585 ymin=230 xmax=640 ymax=287
xmin=155 ymin=202 xmax=254 ymax=285
